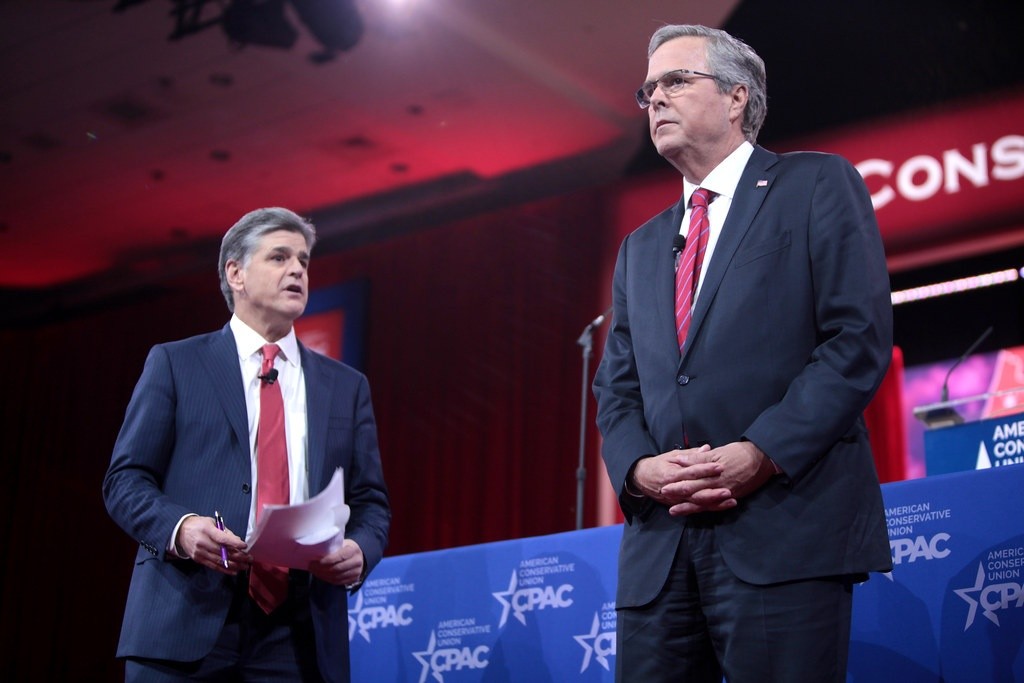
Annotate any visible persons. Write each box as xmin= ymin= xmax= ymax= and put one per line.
xmin=103 ymin=207 xmax=391 ymax=683
xmin=592 ymin=25 xmax=894 ymax=683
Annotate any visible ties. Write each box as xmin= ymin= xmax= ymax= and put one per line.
xmin=248 ymin=344 xmax=291 ymax=616
xmin=676 ymin=188 xmax=718 ymax=447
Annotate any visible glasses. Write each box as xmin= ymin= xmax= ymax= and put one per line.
xmin=635 ymin=69 xmax=733 ymax=110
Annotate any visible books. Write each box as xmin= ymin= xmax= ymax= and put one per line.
xmin=242 ymin=466 xmax=350 ymax=569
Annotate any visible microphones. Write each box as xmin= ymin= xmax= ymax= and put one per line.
xmin=266 ymin=368 xmax=278 ymax=384
xmin=927 ymin=326 xmax=994 ymax=423
xmin=672 ymin=234 xmax=684 ymax=254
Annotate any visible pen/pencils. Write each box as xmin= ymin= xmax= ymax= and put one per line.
xmin=215 ymin=511 xmax=229 ymax=568
